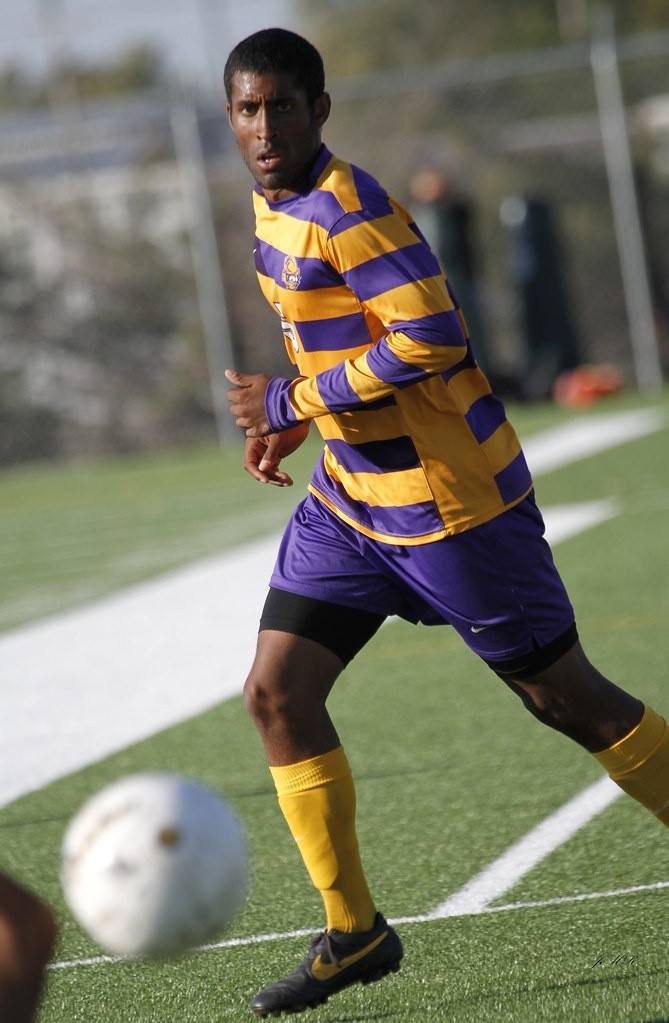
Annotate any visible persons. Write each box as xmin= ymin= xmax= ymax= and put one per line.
xmin=225 ymin=27 xmax=669 ymax=1018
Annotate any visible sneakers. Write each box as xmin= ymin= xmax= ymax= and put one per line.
xmin=250 ymin=911 xmax=403 ymax=1019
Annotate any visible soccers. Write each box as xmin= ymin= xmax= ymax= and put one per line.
xmin=57 ymin=771 xmax=250 ymax=961
